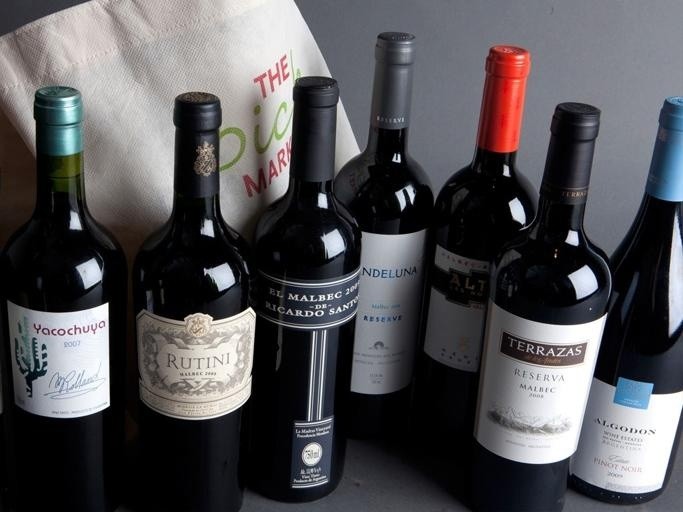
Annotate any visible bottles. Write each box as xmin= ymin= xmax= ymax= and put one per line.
xmin=238 ymin=75 xmax=363 ymax=503
xmin=454 ymin=100 xmax=613 ymax=512
xmin=564 ymin=97 xmax=683 ymax=506
xmin=132 ymin=92 xmax=253 ymax=512
xmin=406 ymin=44 xmax=542 ymax=467
xmin=332 ymin=30 xmax=434 ymax=437
xmin=0 ymin=84 xmax=144 ymax=511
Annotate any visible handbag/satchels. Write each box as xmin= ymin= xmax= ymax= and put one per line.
xmin=0 ymin=0 xmax=362 ymax=309
